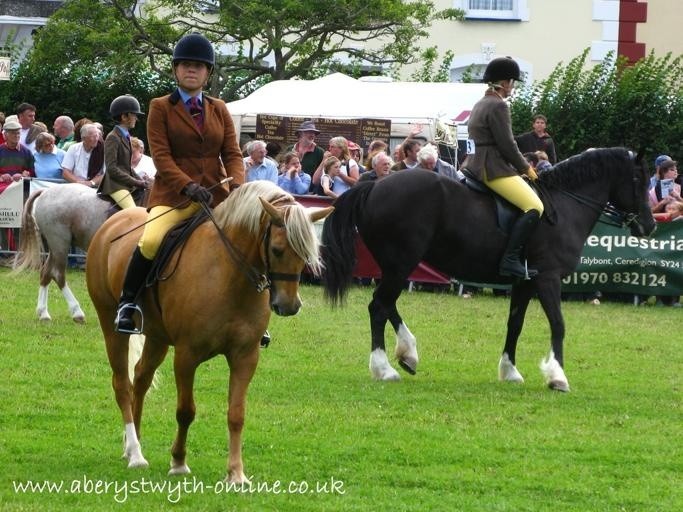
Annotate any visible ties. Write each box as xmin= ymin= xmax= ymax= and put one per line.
xmin=188 ymin=97 xmax=204 ymax=133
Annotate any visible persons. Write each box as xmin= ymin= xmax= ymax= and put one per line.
xmin=0 ymin=30 xmax=683 ymax=345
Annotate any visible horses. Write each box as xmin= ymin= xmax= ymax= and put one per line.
xmin=86 ymin=179 xmax=335 ymax=486
xmin=6 ymin=182 xmax=121 ymax=324
xmin=321 ymin=141 xmax=656 ymax=394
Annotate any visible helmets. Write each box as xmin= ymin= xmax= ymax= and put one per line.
xmin=171 ymin=32 xmax=216 ymax=71
xmin=483 ymin=55 xmax=523 ymax=82
xmin=109 ymin=94 xmax=145 ymax=118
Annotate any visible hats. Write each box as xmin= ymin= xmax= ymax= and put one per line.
xmin=3 ymin=114 xmax=23 ymax=130
xmin=655 ymin=155 xmax=671 ymax=166
xmin=295 ymin=121 xmax=320 ymax=138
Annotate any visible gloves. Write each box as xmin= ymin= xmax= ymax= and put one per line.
xmin=134 ymin=180 xmax=151 ymax=188
xmin=180 ymin=182 xmax=211 ymax=202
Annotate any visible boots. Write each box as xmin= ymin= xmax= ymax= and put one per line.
xmin=498 ymin=209 xmax=540 ymax=278
xmin=113 ymin=246 xmax=153 ymax=335
